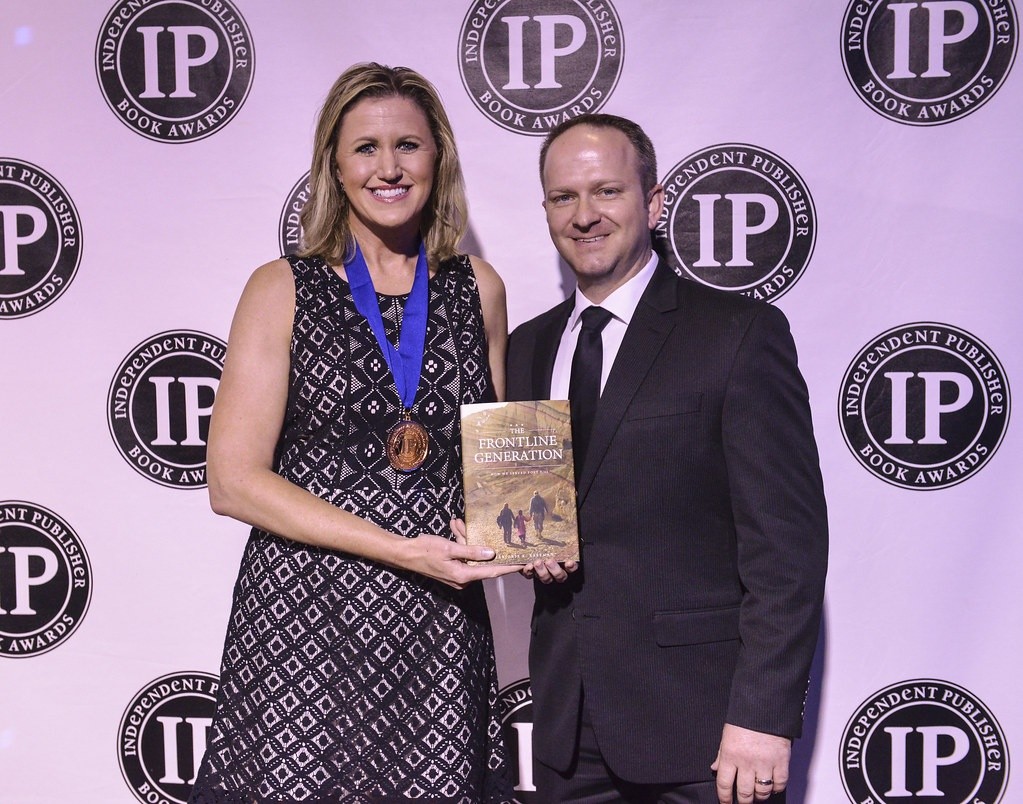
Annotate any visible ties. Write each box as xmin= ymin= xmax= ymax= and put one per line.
xmin=568 ymin=307 xmax=613 ymax=501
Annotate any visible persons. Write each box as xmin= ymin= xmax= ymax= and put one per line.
xmin=514 ymin=510 xmax=532 ymax=543
xmin=505 ymin=114 xmax=829 ymax=804
xmin=187 ymin=60 xmax=526 ymax=804
xmin=529 ymin=490 xmax=549 ymax=538
xmin=497 ymin=502 xmax=516 ymax=547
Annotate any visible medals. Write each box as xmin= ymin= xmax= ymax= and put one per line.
xmin=386 ymin=416 xmax=429 ymax=473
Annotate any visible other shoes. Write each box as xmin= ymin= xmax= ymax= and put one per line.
xmin=505 ymin=542 xmax=514 ymax=546
xmin=536 ymin=535 xmax=543 ymax=539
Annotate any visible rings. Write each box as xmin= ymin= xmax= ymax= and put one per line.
xmin=755 ymin=778 xmax=774 ymax=785
